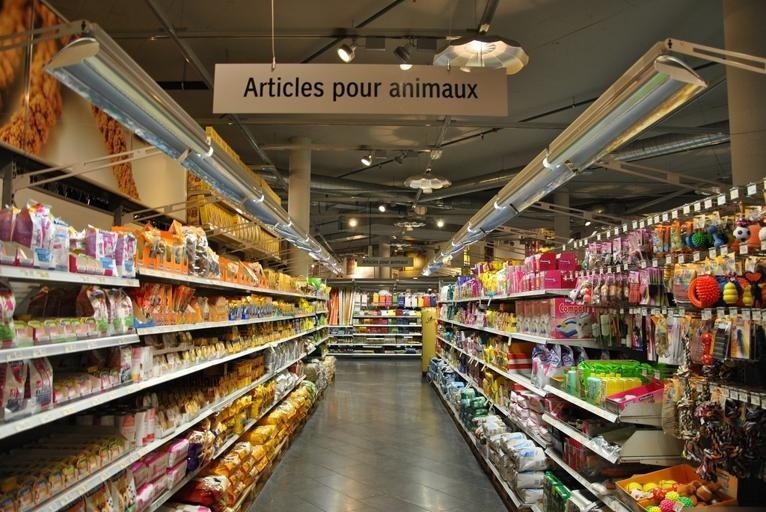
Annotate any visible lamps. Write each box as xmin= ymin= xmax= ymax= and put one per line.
xmin=433 ymin=34 xmax=527 ymax=76
xmin=360 ymin=151 xmax=409 ymax=167
xmin=332 ymin=35 xmax=423 ymax=63
xmin=403 ymin=171 xmax=453 ymax=193
xmin=393 ymin=218 xmax=425 ymax=230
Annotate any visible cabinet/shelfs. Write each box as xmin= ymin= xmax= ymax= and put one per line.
xmin=544 ymin=381 xmax=686 ymax=512
xmin=0 ymin=258 xmax=328 ymax=512
xmin=328 ymin=304 xmax=425 ymax=358
xmin=427 ymin=288 xmax=578 ymax=508
